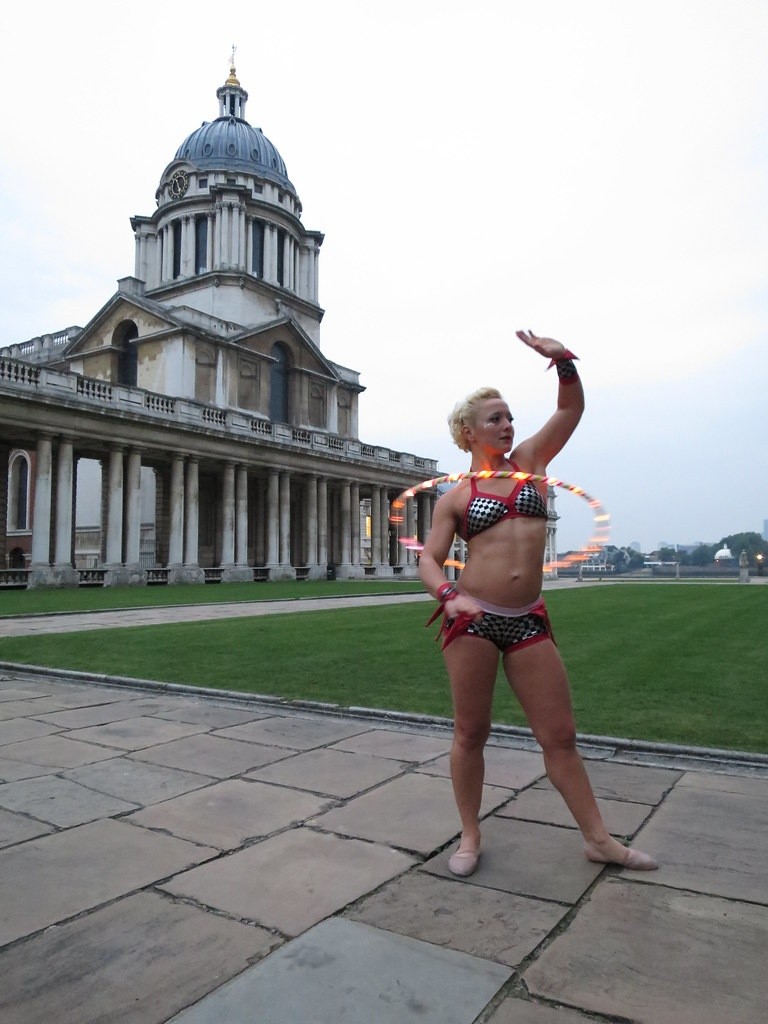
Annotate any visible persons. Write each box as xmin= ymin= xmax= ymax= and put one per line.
xmin=416 ymin=329 xmax=661 ymax=877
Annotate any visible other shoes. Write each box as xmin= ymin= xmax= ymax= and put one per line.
xmin=584 ymin=842 xmax=658 ymax=870
xmin=448 ymin=844 xmax=482 ymax=876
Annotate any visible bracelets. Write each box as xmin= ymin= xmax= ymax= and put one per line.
xmin=436 ymin=582 xmax=460 ymax=602
xmin=547 ymin=350 xmax=580 ymax=384
